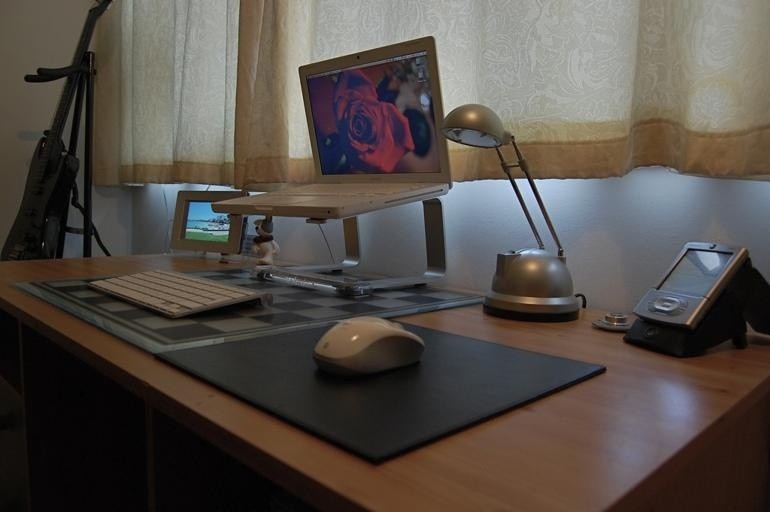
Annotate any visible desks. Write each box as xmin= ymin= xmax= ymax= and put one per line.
xmin=1 ymin=250 xmax=769 ymax=511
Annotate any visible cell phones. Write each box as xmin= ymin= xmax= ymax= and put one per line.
xmin=633 ymin=242 xmax=749 ymax=329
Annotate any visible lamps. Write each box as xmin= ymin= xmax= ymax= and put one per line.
xmin=440 ymin=105 xmax=579 ymax=321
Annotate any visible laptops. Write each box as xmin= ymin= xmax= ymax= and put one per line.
xmin=212 ymin=35 xmax=452 ymax=219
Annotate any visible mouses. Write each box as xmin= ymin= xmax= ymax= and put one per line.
xmin=313 ymin=316 xmax=425 ymax=378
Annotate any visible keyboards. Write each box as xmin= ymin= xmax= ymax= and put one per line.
xmin=90 ymin=269 xmax=273 ymax=319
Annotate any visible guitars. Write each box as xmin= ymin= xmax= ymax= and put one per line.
xmin=0 ymin=0 xmax=110 ymax=261
